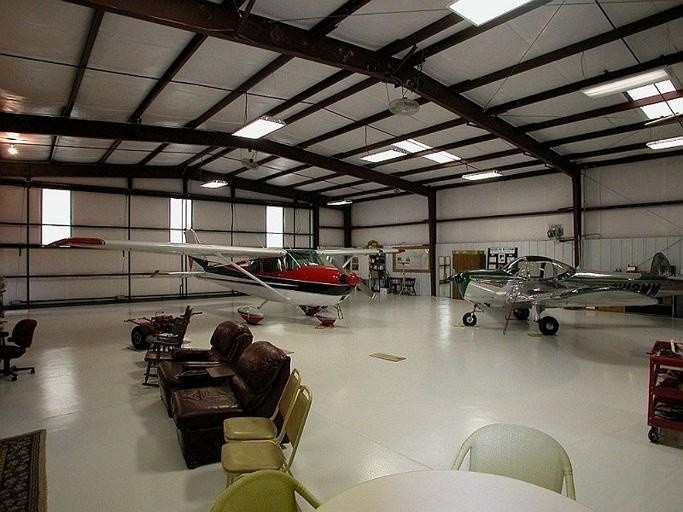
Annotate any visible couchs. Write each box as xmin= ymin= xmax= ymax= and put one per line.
xmin=157 ymin=320 xmax=253 ymax=417
xmin=171 ymin=342 xmax=290 ymax=468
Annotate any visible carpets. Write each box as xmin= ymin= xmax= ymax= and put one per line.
xmin=0 ymin=428 xmax=46 ymax=511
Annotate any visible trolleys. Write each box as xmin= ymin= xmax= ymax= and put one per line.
xmin=123 ymin=306 xmax=202 ymax=348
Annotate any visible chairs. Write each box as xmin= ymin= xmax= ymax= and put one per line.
xmin=1 ymin=317 xmax=37 ymax=381
xmin=222 ymin=367 xmax=301 ymax=440
xmin=210 ymin=468 xmax=320 ymax=511
xmin=142 ymin=334 xmax=183 ymax=386
xmin=221 ymin=385 xmax=312 ymax=486
xmin=452 ymin=423 xmax=575 ymax=498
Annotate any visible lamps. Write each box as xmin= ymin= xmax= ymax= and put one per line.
xmin=240 ymin=150 xmax=260 ymax=171
xmin=389 ymin=82 xmax=420 ymax=117
